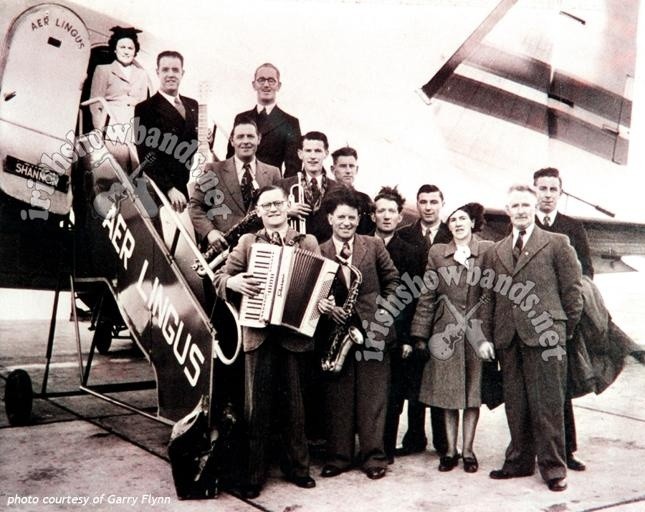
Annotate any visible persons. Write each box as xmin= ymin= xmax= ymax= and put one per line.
xmin=133 ymin=48 xmax=199 ymax=213
xmin=395 ymin=184 xmax=454 ymax=455
xmin=89 ymin=25 xmax=155 ymax=183
xmin=410 ymin=198 xmax=495 ymax=474
xmin=187 ymin=116 xmax=425 ymax=499
xmin=533 ymin=167 xmax=594 ymax=471
xmin=474 ymin=185 xmax=584 ymax=491
xmin=227 ymin=63 xmax=303 ymax=178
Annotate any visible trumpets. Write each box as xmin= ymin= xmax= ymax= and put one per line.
xmin=288 ymin=171 xmax=307 ymax=236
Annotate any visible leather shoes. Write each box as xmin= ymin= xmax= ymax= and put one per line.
xmin=290 ymin=446 xmax=587 ymax=491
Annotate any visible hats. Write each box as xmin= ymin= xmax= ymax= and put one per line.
xmin=440 ymin=200 xmax=485 ymax=223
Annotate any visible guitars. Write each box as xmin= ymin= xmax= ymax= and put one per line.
xmin=187 ymin=80 xmax=214 ymax=201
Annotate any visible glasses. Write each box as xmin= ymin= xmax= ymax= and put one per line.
xmin=261 ymin=200 xmax=283 ymax=211
xmin=256 ymin=76 xmax=277 ymax=85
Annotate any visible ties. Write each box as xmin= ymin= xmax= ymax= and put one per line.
xmin=258 ymin=107 xmax=267 ymax=127
xmin=514 ymin=229 xmax=525 ymax=265
xmin=543 ymin=216 xmax=550 ymax=223
xmin=426 ymin=229 xmax=432 ymax=251
xmin=243 ymin=165 xmax=251 ymax=185
xmin=310 ymin=178 xmax=318 ymax=207
xmin=176 ymin=97 xmax=186 ymax=119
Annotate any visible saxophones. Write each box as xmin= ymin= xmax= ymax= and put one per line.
xmin=195 ymin=207 xmax=260 ymax=278
xmin=321 ymin=255 xmax=364 ymax=372
